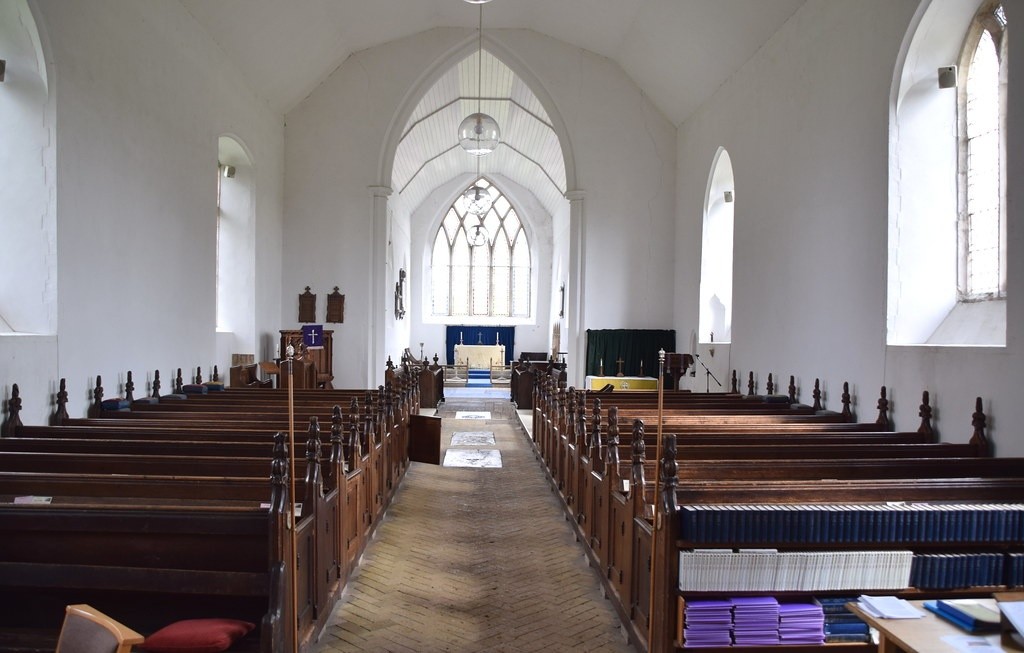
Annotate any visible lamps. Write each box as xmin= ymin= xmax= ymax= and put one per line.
xmin=468 ymin=225 xmax=488 ymax=246
xmin=463 ymin=155 xmax=492 ymax=215
xmin=458 ymin=2 xmax=501 ymax=157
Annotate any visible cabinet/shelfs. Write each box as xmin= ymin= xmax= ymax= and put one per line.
xmin=650 ymin=501 xmax=1024 ymax=653
xmin=279 ymin=328 xmax=335 ymax=390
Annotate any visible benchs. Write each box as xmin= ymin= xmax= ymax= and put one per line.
xmin=504 ymin=368 xmax=1024 ymax=640
xmin=0 ymin=362 xmax=443 ymax=653
xmin=405 ymin=348 xmax=423 ymax=369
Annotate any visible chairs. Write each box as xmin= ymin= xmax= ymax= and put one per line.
xmin=56 ymin=603 xmax=145 ymax=653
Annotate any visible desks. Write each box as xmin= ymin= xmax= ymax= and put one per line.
xmin=454 ymin=344 xmax=506 ymax=370
xmin=509 ymin=360 xmax=567 ymax=373
xmin=844 ymin=598 xmax=1024 ymax=652
xmin=585 ymin=376 xmax=658 ymax=389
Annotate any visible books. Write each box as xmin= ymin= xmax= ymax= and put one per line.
xmin=682 ymin=596 xmax=873 ymax=649
xmin=922 ymin=598 xmax=1003 ymax=635
xmin=680 ymin=548 xmax=1024 ymax=591
xmin=681 ymin=501 xmax=1024 ymax=545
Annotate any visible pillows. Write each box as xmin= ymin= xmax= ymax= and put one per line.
xmin=136 ymin=618 xmax=257 ymax=653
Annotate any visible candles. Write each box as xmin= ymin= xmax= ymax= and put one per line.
xmin=641 ymin=360 xmax=643 ymax=367
xmin=497 ymin=332 xmax=499 ymax=340
xmin=460 ymin=331 xmax=462 ymax=340
xmin=601 ymin=357 xmax=603 ymax=366
